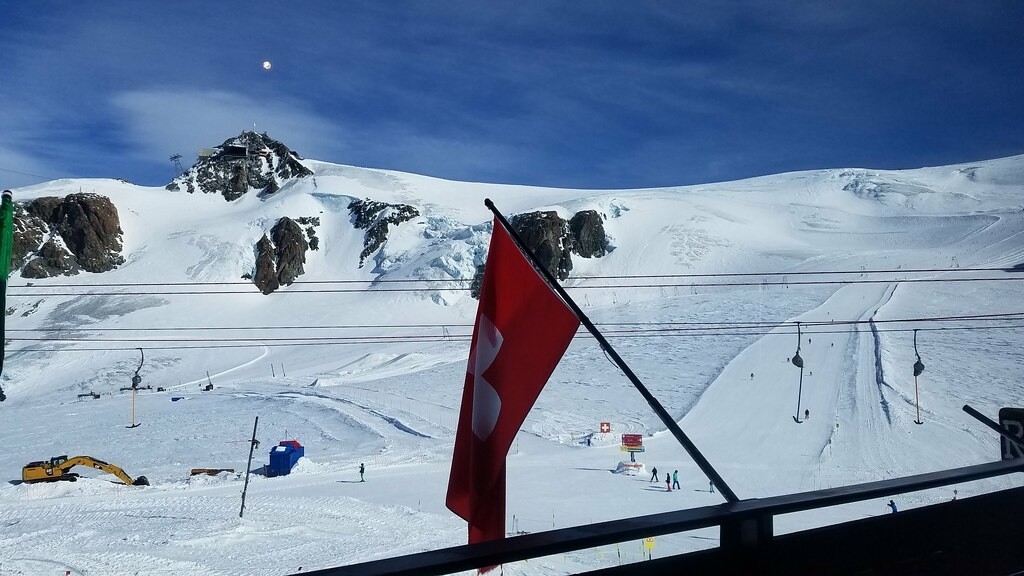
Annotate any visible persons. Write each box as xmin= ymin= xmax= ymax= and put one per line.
xmin=673 ymin=470 xmax=681 ymax=489
xmin=809 ymin=339 xmax=811 ymax=343
xmin=787 ymin=358 xmax=789 ymax=362
xmin=751 ymin=373 xmax=755 ymax=380
xmin=665 ymin=473 xmax=672 ymax=491
xmin=710 ymin=482 xmax=714 ymax=493
xmin=953 ymin=490 xmax=960 ymax=500
xmin=805 ymin=408 xmax=810 ymax=419
xmin=358 ymin=463 xmax=366 ymax=482
xmin=810 ymin=372 xmax=812 ymax=375
xmin=651 ymin=467 xmax=659 ymax=482
xmin=887 ymin=500 xmax=897 ymax=513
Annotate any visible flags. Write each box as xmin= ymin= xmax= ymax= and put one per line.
xmin=444 ymin=218 xmax=581 ymax=576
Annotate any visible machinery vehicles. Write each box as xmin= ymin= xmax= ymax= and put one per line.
xmin=22 ymin=455 xmax=150 ymax=486
